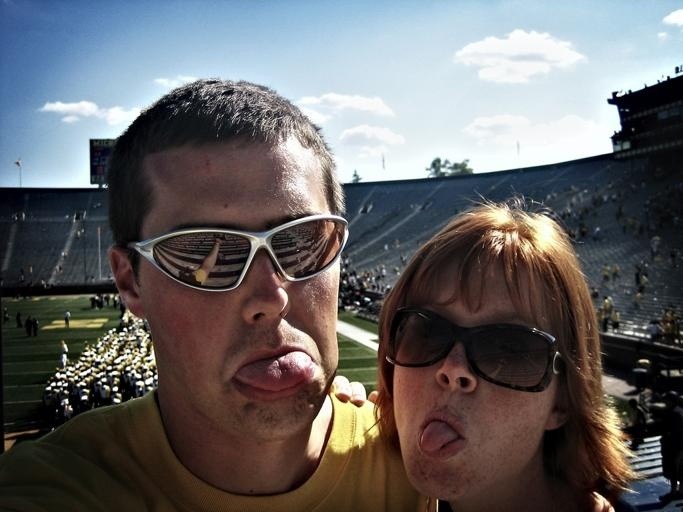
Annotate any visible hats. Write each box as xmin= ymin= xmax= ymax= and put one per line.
xmin=45 ymin=310 xmax=158 ymax=404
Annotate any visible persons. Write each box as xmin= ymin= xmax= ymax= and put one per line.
xmin=1 ymin=79 xmax=438 ymax=509
xmin=2 ymin=167 xmax=682 ymax=510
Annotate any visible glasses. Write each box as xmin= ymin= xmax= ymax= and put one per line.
xmin=385 ymin=307 xmax=561 ymax=393
xmin=127 ymin=214 xmax=350 ymax=291
